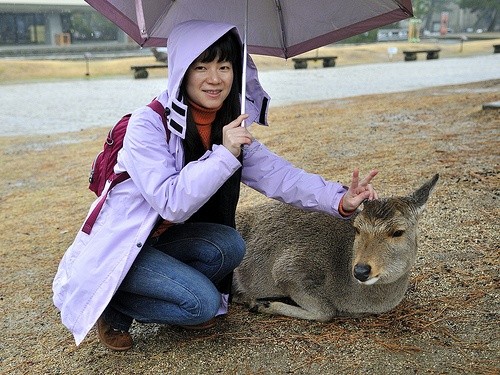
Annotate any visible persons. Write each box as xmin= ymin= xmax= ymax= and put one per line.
xmin=52 ymin=18 xmax=380 ymax=352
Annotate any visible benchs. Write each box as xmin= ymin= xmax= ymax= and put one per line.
xmin=131 ymin=65 xmax=168 ymax=78
xmin=292 ymin=56 xmax=337 ymax=69
xmin=403 ymin=49 xmax=441 ymax=61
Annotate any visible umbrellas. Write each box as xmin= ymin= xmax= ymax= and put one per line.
xmin=84 ymin=0 xmax=418 ymax=127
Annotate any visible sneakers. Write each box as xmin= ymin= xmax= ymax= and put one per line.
xmin=97 ymin=317 xmax=133 ymax=350
xmin=181 ymin=320 xmax=214 ymax=329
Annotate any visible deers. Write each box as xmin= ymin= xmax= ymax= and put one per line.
xmin=228 ymin=173 xmax=443 ymax=324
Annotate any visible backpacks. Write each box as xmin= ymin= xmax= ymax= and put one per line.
xmin=81 ymin=97 xmax=171 ymax=235
